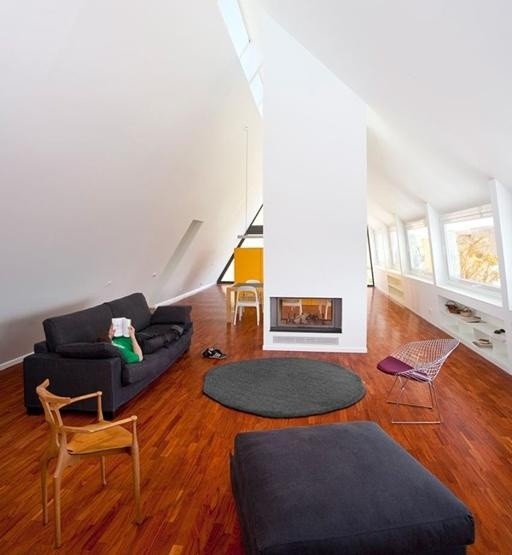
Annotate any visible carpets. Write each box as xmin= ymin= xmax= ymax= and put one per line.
xmin=203 ymin=357 xmax=367 ymax=417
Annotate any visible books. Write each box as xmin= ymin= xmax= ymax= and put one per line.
xmin=112 ymin=318 xmax=131 ymax=337
xmin=473 ymin=341 xmax=493 ymax=348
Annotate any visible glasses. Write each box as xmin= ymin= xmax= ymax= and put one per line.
xmin=495 ymin=329 xmax=506 ymax=334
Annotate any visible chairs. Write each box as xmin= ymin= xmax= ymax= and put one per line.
xmin=234 ymin=279 xmax=263 ymax=327
xmin=378 ymin=338 xmax=460 ymax=424
xmin=34 ymin=378 xmax=142 ymax=548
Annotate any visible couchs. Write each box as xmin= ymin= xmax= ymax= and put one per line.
xmin=23 ymin=293 xmax=193 ymax=418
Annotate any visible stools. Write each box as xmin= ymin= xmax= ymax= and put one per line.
xmin=231 ymin=421 xmax=474 ymax=555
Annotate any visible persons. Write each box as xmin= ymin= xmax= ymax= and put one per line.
xmin=288 ymin=307 xmax=296 ymax=323
xmin=95 ymin=320 xmax=193 ymax=363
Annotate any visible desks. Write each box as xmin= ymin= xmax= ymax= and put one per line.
xmin=226 ymin=283 xmax=264 ymax=323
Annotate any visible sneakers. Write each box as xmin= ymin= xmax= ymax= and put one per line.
xmin=203 ymin=348 xmax=227 ymax=360
